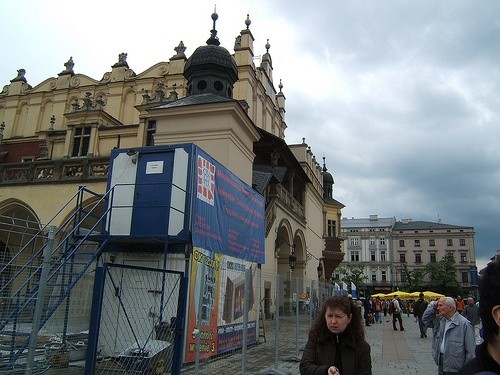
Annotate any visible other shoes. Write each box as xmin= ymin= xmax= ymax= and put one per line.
xmin=421 ymin=336 xmax=424 ymax=338
xmin=394 ymin=328 xmax=398 ymax=330
xmin=366 ymin=324 xmax=370 ymax=326
xmin=401 ymin=329 xmax=407 ymax=331
xmin=424 ymin=333 xmax=427 ymax=338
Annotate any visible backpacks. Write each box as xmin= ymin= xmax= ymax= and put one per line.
xmin=388 ymin=300 xmax=397 ymax=315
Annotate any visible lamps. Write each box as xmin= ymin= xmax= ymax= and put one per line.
xmin=318 ymin=260 xmax=323 ymax=277
xmin=275 ymin=233 xmax=296 ymax=271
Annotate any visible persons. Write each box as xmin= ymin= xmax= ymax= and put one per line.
xmin=299 ymin=295 xmax=373 ymax=375
xmin=349 ymin=294 xmax=481 ymax=375
xmin=459 ymin=259 xmax=500 ymax=375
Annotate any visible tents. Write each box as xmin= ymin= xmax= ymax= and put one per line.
xmin=374 ymin=291 xmax=445 ymax=304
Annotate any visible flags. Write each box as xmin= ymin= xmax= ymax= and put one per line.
xmin=335 ymin=282 xmax=359 ymax=300
